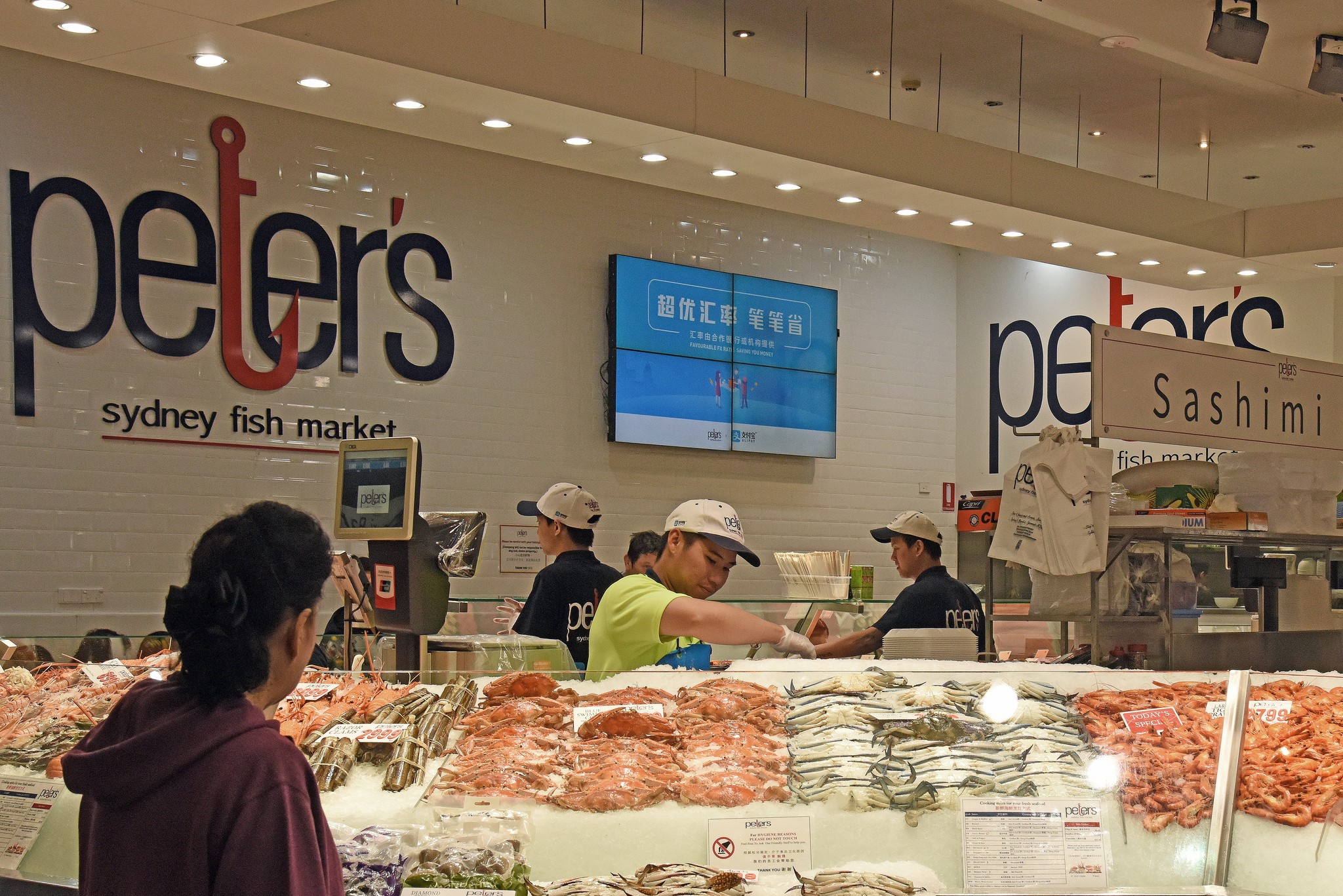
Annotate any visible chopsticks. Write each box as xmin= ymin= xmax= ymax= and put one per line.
xmin=775 ymin=550 xmax=851 ymax=598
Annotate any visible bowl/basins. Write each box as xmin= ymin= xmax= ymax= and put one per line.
xmin=1213 ymin=597 xmax=1238 ymax=608
xmin=780 ymin=574 xmax=852 ymax=600
xmin=1297 ymin=557 xmax=1317 ymax=576
xmin=1111 ymin=460 xmax=1219 ymax=494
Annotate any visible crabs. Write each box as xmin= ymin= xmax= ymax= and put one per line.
xmin=522 ymin=860 xmax=928 ymax=896
xmin=430 ymin=670 xmax=1077 ymax=828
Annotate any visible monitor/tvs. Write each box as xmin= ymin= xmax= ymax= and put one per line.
xmin=333 ymin=436 xmax=422 ymax=540
xmin=418 ymin=512 xmax=487 ymax=578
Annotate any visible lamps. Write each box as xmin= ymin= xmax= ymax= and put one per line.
xmin=1206 ymin=0 xmax=1269 ymax=65
xmin=1308 ymin=34 xmax=1343 ymax=96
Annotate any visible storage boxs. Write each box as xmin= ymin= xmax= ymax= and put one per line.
xmin=1108 ymin=515 xmax=1183 ymax=528
xmin=778 ymin=565 xmax=874 ymax=599
xmin=1173 ymin=609 xmax=1203 ymax=634
xmin=1209 ymin=512 xmax=1268 ymax=531
xmin=1135 ymin=509 xmax=1206 ymax=529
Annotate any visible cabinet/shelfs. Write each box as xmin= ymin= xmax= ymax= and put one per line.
xmin=961 ymin=499 xmax=1172 ymax=673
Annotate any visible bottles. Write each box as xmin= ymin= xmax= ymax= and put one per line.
xmin=1127 ymin=644 xmax=1148 ymax=670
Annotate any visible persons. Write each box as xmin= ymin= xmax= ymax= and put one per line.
xmin=60 ymin=501 xmax=348 ymax=896
xmin=137 ymin=631 xmax=179 ymax=660
xmin=584 ymin=499 xmax=817 ymax=684
xmin=68 ymin=628 xmax=132 ymax=669
xmin=1191 ymin=559 xmax=1210 ymax=610
xmin=788 ymin=510 xmax=998 ymax=661
xmin=809 ymin=618 xmax=830 ymax=646
xmin=2 ymin=645 xmax=58 ymax=676
xmin=620 ymin=530 xmax=662 ymax=577
xmin=319 ymin=556 xmax=383 ymax=678
xmin=493 ymin=482 xmax=625 ymax=678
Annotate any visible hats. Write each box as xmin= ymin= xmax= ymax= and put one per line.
xmin=516 ymin=483 xmax=600 ymax=530
xmin=665 ymin=499 xmax=762 ymax=568
xmin=869 ymin=510 xmax=943 ymax=544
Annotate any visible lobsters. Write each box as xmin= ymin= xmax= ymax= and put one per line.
xmin=0 ymin=627 xmax=420 ymax=781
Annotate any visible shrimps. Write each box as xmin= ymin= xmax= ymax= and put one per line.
xmin=1077 ymin=676 xmax=1343 ymax=834
